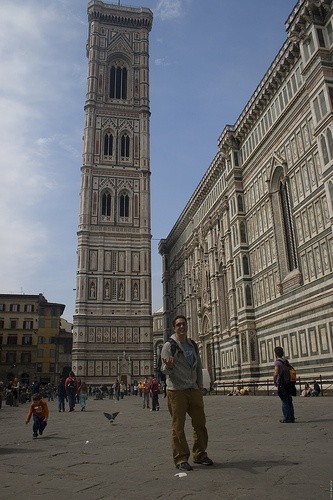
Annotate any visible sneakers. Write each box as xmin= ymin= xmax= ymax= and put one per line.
xmin=176 ymin=462 xmax=193 ymax=470
xmin=202 ymin=457 xmax=213 ymax=464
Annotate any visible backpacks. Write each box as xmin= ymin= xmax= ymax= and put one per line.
xmin=159 ymin=338 xmax=199 ymax=381
xmin=277 ymin=360 xmax=296 ymax=382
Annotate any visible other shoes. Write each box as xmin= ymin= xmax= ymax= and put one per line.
xmin=280 ymin=420 xmax=294 ymax=423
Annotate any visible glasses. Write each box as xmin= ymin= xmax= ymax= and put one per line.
xmin=174 ymin=322 xmax=187 ymax=327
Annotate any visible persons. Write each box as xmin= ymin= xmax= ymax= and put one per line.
xmin=65 ymin=370 xmax=79 ymax=412
xmin=161 ymin=315 xmax=213 ymax=471
xmin=274 ymin=347 xmax=296 ymax=423
xmin=112 ymin=378 xmax=120 ymax=401
xmin=26 ymin=393 xmax=49 ymax=439
xmin=57 ymin=373 xmax=66 ymax=412
xmin=89 ymin=383 xmax=113 ymax=400
xmin=301 ymin=380 xmax=320 ymax=397
xmin=139 ymin=374 xmax=160 ymax=412
xmin=78 ymin=381 xmax=87 ymax=411
xmin=119 ymin=380 xmax=125 ymax=399
xmin=0 ymin=380 xmax=56 ymax=409
xmin=127 ymin=383 xmax=138 ymax=396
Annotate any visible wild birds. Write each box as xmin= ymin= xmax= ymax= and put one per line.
xmin=103 ymin=411 xmax=120 ymax=423
